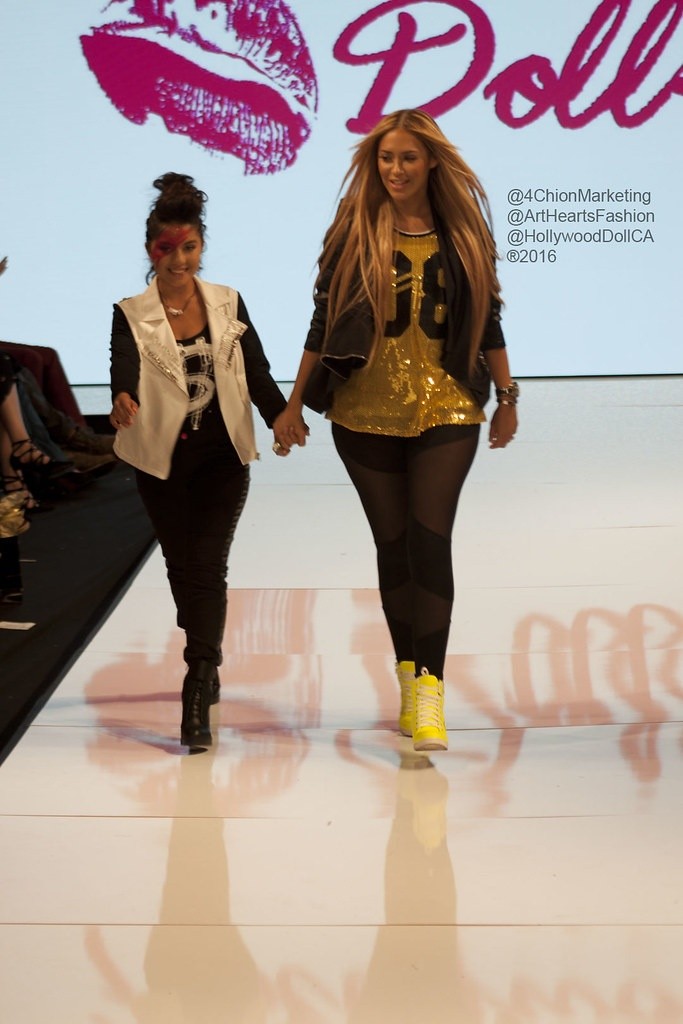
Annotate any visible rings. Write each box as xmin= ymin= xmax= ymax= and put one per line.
xmin=273 ymin=442 xmax=281 ymax=454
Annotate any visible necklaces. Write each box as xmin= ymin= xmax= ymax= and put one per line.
xmin=158 ymin=285 xmax=196 ymax=316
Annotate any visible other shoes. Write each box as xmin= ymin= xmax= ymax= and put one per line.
xmin=65 ymin=449 xmax=118 ymax=477
xmin=66 ymin=427 xmax=123 ymax=455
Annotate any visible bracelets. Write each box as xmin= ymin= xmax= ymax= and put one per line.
xmin=496 ymin=382 xmax=519 ymax=406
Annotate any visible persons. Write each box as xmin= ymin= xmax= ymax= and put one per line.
xmin=0 ymin=256 xmax=122 ymax=608
xmin=109 ymin=171 xmax=310 ymax=750
xmin=272 ymin=109 xmax=520 ymax=752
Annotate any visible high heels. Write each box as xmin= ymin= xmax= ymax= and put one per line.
xmin=9 ymin=439 xmax=74 ymax=484
xmin=409 ymin=667 xmax=447 ymax=752
xmin=395 ymin=660 xmax=418 ymax=737
xmin=1 ymin=468 xmax=54 ymax=515
xmin=210 ymin=664 xmax=221 ymax=706
xmin=178 ymin=660 xmax=213 ymax=751
